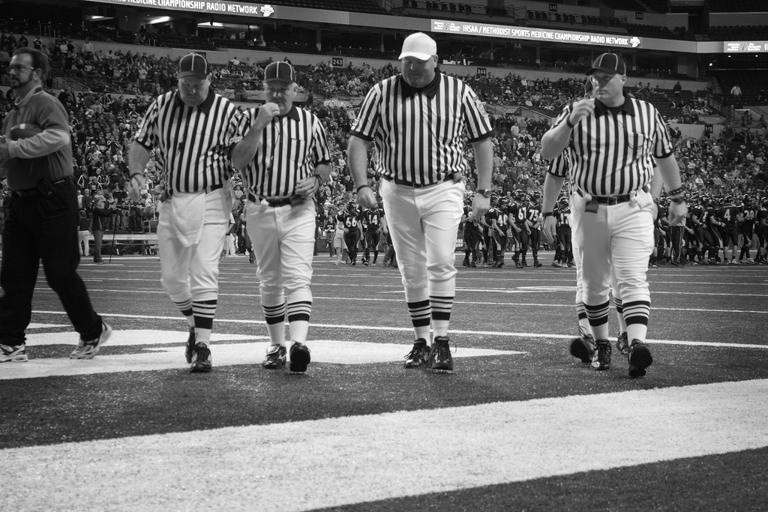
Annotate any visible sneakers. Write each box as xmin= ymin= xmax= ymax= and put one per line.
xmin=0 ymin=343 xmax=28 ymax=363
xmin=570 ymin=332 xmax=652 ymax=376
xmin=69 ymin=321 xmax=111 ymax=359
xmin=264 ymin=342 xmax=310 ymax=372
xmin=186 ymin=326 xmax=211 ymax=372
xmin=405 ymin=336 xmax=453 ymax=373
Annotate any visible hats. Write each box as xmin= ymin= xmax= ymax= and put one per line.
xmin=262 ymin=61 xmax=297 ymax=84
xmin=176 ymin=52 xmax=209 ymax=79
xmin=397 ymin=32 xmax=437 ymax=61
xmin=585 ymin=52 xmax=626 ymax=75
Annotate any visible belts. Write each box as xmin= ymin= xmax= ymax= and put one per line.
xmin=248 ymin=193 xmax=290 ymax=207
xmin=160 ymin=184 xmax=223 ymax=203
xmin=383 ymin=174 xmax=454 ymax=188
xmin=577 ymin=188 xmax=630 ymax=205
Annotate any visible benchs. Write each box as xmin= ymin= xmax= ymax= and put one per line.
xmin=114 ymin=242 xmax=157 ymax=255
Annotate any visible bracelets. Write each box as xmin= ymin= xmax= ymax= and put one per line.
xmin=669 ymin=187 xmax=685 ymax=193
xmin=355 ymin=184 xmax=369 ymax=192
xmin=130 ymin=173 xmax=141 ymax=180
xmin=542 ymin=212 xmax=554 ymax=218
xmin=313 ymin=174 xmax=324 ymax=187
xmin=566 ymin=113 xmax=574 ymax=130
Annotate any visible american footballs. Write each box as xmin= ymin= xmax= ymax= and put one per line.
xmin=10 ymin=122 xmax=41 ymax=140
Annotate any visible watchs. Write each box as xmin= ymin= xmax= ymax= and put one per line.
xmin=670 ymin=196 xmax=687 ymax=203
xmin=478 ymin=188 xmax=493 ymax=200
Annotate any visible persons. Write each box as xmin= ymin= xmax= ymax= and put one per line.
xmin=348 ymin=32 xmax=495 ymax=370
xmin=542 ymin=52 xmax=689 ymax=378
xmin=230 ymin=60 xmax=332 ymax=372
xmin=0 ymin=47 xmax=112 ymax=363
xmin=130 ymin=54 xmax=243 ymax=371
xmin=0 ymin=14 xmax=768 ymax=267
xmin=541 ymin=75 xmax=664 ymax=363
